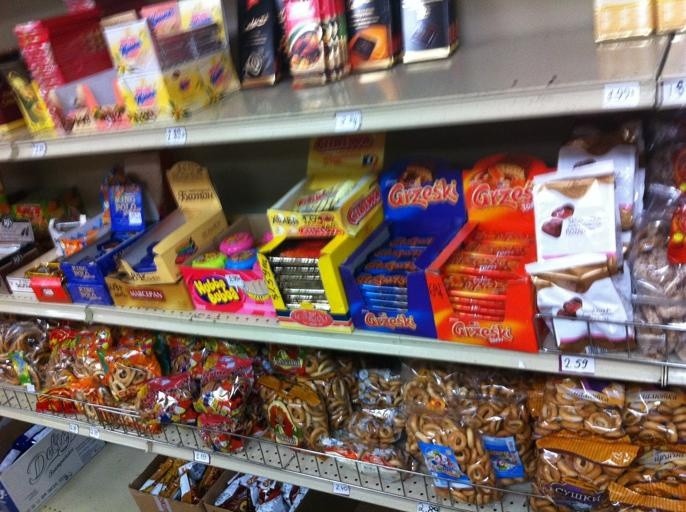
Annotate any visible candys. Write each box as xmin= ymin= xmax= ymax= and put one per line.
xmin=426 ymin=219 xmax=538 ymax=353
xmin=28 ymin=249 xmax=72 ymax=304
xmin=266 ymin=236 xmax=345 ymax=320
xmin=347 ymin=217 xmax=436 ymax=338
xmin=274 ymin=174 xmax=371 ymax=238
xmin=117 ymin=207 xmax=179 ymax=284
xmin=62 ymin=226 xmax=139 ymax=308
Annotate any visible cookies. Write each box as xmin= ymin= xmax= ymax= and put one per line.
xmin=323 ymin=367 xmax=417 ymax=480
xmin=255 ymin=342 xmax=358 ymax=458
xmin=137 ymin=333 xmax=269 ymax=455
xmin=528 ymin=374 xmax=686 ymax=511
xmin=0 ymin=313 xmax=49 ymax=392
xmin=402 ymin=357 xmax=537 ymax=503
xmin=35 ymin=324 xmax=173 ymax=436
